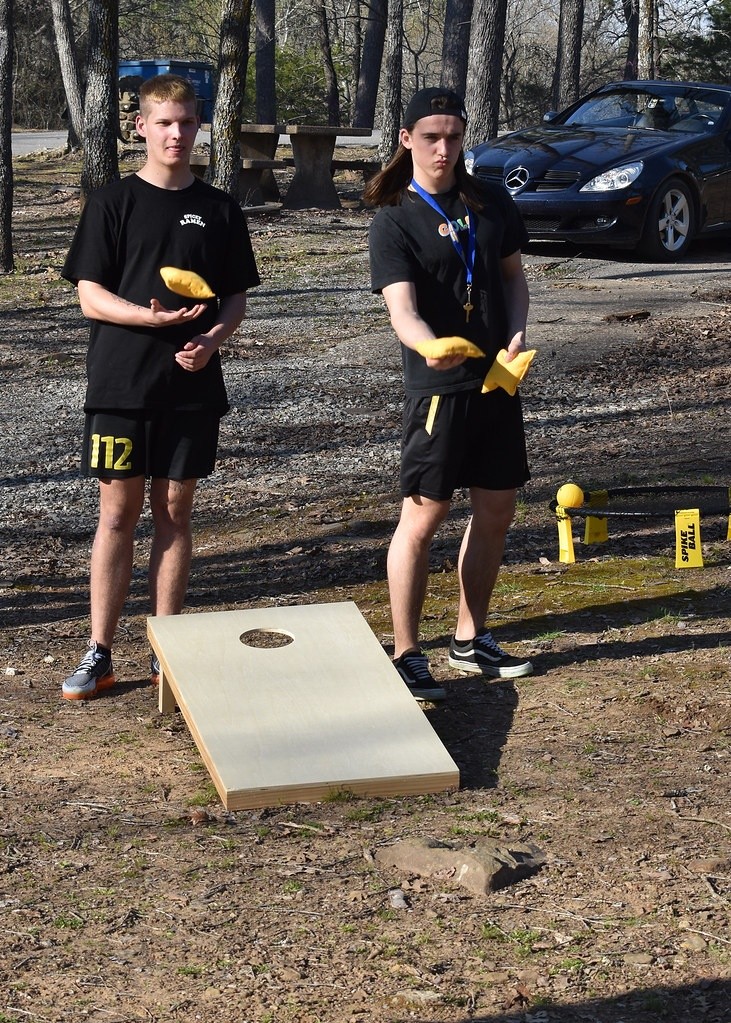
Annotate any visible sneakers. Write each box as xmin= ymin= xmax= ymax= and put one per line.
xmin=450 ymin=633 xmax=534 ymax=679
xmin=62 ymin=651 xmax=115 ymax=700
xmin=149 ymin=655 xmax=162 ymax=686
xmin=390 ymin=651 xmax=448 ymax=701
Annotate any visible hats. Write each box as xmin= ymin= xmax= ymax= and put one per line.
xmin=402 ymin=88 xmax=467 ymax=128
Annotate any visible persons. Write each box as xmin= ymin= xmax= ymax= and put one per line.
xmin=358 ymin=87 xmax=537 ymax=703
xmin=56 ymin=75 xmax=261 ymax=704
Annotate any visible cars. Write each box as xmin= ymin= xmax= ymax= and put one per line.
xmin=464 ymin=79 xmax=731 ymax=267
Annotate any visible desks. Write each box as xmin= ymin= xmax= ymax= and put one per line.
xmin=200 ymin=120 xmax=372 ymax=215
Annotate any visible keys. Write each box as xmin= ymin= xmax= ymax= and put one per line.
xmin=463 ymin=301 xmax=474 ymax=323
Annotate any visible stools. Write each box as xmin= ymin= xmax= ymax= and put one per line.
xmin=283 ymin=157 xmax=383 ymax=210
xmin=190 ymin=154 xmax=288 ymax=212
